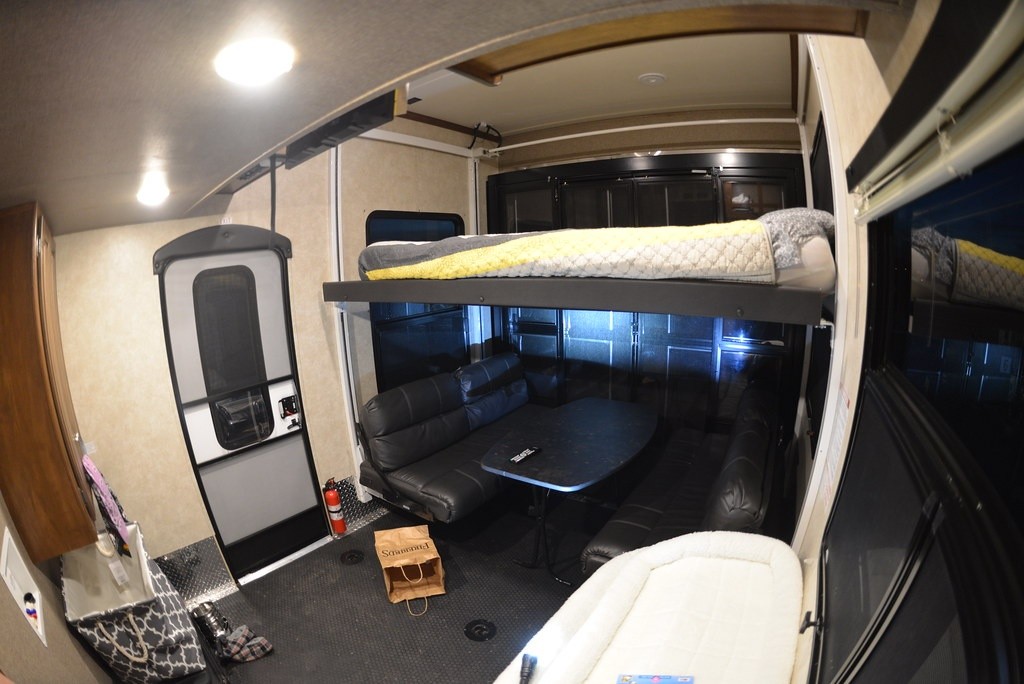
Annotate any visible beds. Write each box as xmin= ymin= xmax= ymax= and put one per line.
xmin=912 ymin=226 xmax=1024 ymax=308
xmin=356 ymin=205 xmax=835 ymax=296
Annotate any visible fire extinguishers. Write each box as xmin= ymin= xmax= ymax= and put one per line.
xmin=322 ymin=476 xmax=350 ymax=538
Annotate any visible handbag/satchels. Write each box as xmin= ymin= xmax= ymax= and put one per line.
xmin=372 ymin=524 xmax=447 ymax=616
xmin=63 ymin=522 xmax=206 ymax=683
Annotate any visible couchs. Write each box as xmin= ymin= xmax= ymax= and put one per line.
xmin=358 ymin=350 xmax=782 ymax=578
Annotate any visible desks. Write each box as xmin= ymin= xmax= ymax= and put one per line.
xmin=481 ymin=394 xmax=659 ymax=587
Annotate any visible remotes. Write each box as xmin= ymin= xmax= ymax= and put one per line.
xmin=510 ymin=447 xmax=540 ymax=464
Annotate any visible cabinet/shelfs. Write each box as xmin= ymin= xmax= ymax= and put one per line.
xmin=0 ymin=201 xmax=100 ymax=563
